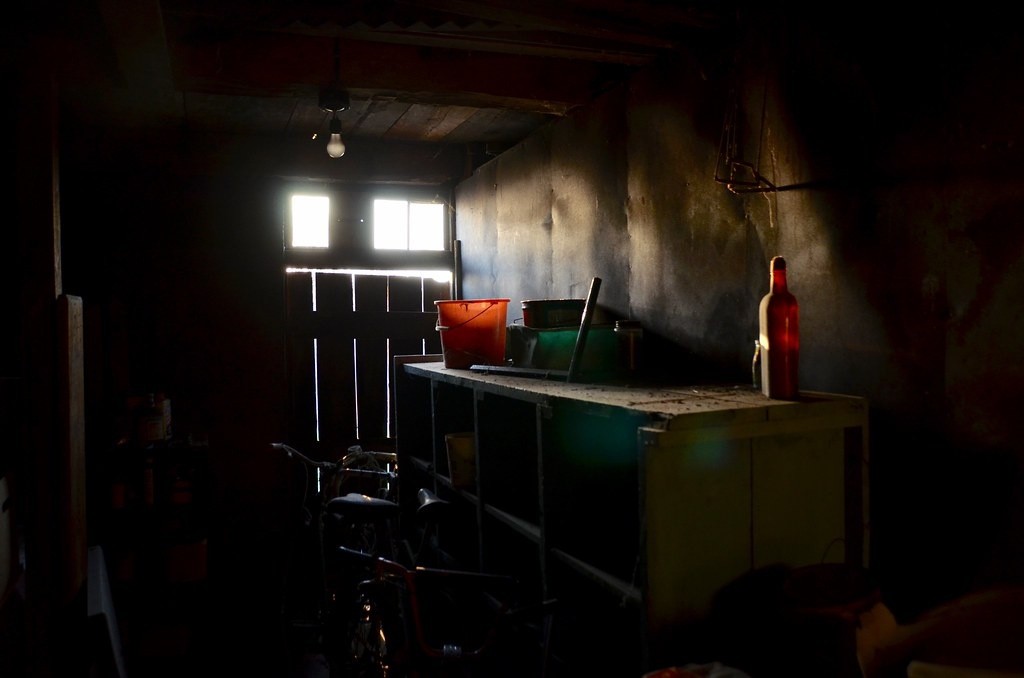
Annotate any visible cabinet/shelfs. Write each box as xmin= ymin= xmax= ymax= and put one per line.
xmin=391 ymin=352 xmax=871 ymax=678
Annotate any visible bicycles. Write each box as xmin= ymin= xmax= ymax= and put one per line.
xmin=266 ymin=438 xmax=522 ymax=678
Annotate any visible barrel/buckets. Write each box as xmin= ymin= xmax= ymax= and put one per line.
xmin=522 ymin=299 xmax=586 ymax=329
xmin=434 ymin=298 xmax=511 ymax=370
xmin=444 ymin=432 xmax=476 ymax=490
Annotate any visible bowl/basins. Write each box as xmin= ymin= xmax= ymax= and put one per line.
xmin=507 ymin=325 xmax=620 ymax=379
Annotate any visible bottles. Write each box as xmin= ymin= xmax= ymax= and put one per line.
xmin=758 ymin=256 xmax=801 ymax=399
xmin=141 ymin=389 xmax=175 ymax=444
